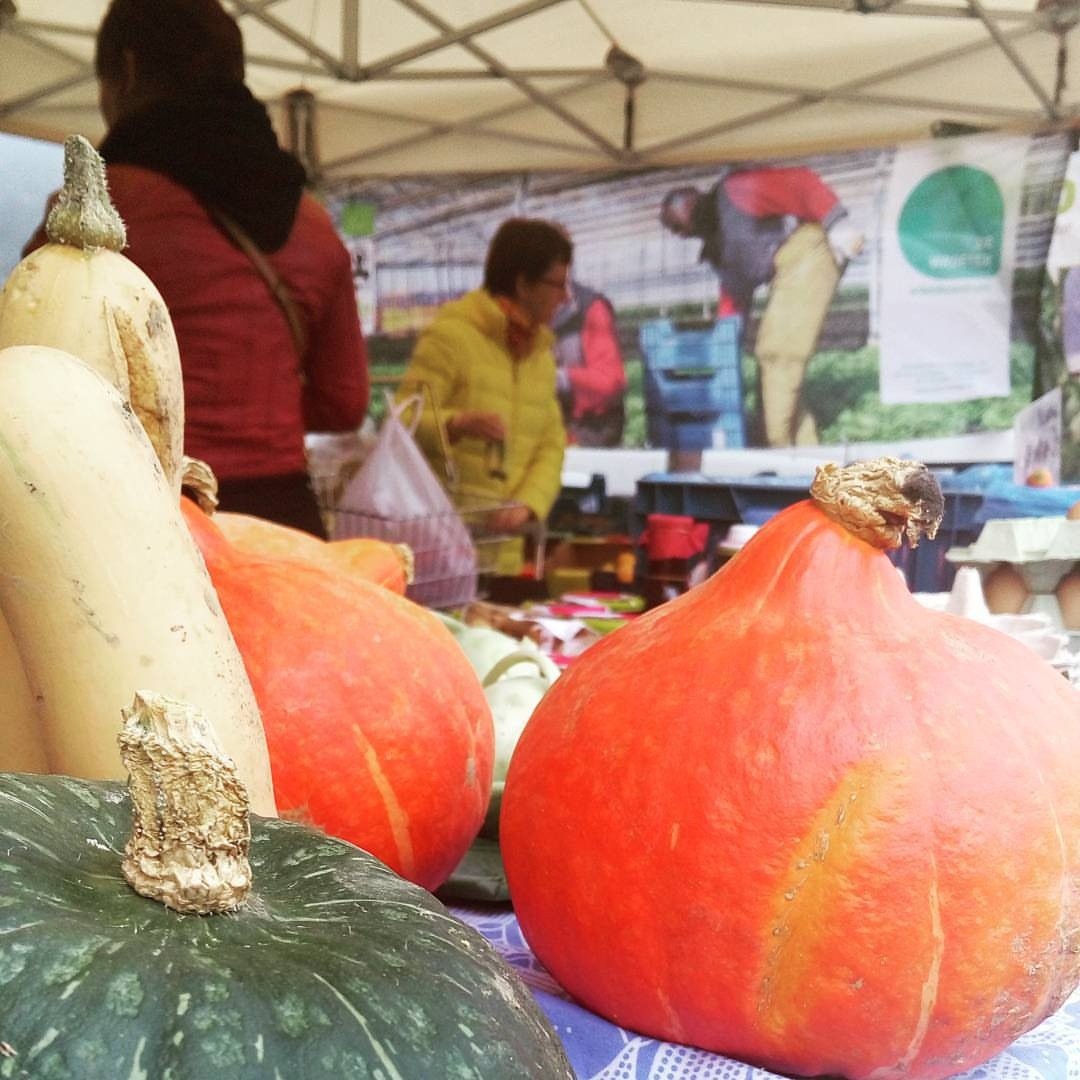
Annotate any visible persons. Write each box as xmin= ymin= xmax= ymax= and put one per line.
xmin=550 ymin=280 xmax=630 ymax=448
xmin=16 ymin=0 xmax=369 ymax=546
xmin=387 ymin=211 xmax=568 ymax=603
xmin=660 ymin=163 xmax=866 ymax=448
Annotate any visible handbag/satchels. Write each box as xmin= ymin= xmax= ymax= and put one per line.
xmin=330 ymin=389 xmax=480 ymax=609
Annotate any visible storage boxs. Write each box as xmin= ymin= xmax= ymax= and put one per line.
xmin=637 ymin=314 xmax=748 ymax=453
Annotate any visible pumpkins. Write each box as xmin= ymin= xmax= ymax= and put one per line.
xmin=3 ymin=131 xmax=494 ymax=896
xmin=501 ymin=465 xmax=1078 ymax=1080
xmin=1 ymin=689 xmax=575 ymax=1078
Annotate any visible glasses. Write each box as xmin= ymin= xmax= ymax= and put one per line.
xmin=538 ymin=278 xmax=567 ymax=291
